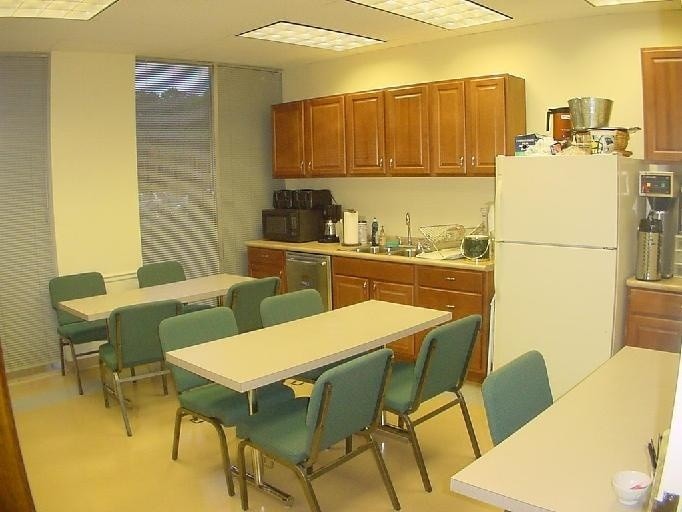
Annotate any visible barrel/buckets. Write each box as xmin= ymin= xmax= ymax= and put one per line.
xmin=460 ymin=234 xmax=491 ymax=265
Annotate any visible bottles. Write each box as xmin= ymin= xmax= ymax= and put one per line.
xmin=379 ymin=225 xmax=387 ymax=245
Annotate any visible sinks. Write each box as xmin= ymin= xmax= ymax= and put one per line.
xmin=347 ymin=243 xmax=402 ymax=255
xmin=384 ymin=246 xmax=431 ymax=258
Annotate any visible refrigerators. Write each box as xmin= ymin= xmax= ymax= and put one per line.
xmin=490 ymin=154 xmax=644 ymax=402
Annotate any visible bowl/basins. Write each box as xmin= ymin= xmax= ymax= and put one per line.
xmin=611 ymin=470 xmax=653 ymax=506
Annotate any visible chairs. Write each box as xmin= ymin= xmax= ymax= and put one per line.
xmin=344 ymin=312 xmax=485 ymax=493
xmin=257 ymin=288 xmax=370 ymax=390
xmin=137 ymin=258 xmax=215 ymax=317
xmin=155 ymin=305 xmax=296 ymax=497
xmin=233 ymin=347 xmax=402 ymax=512
xmin=96 ymin=299 xmax=184 ymax=438
xmin=47 ymin=270 xmax=137 ymax=398
xmin=480 ymin=349 xmax=555 ymax=447
xmin=223 ymin=272 xmax=281 ymax=335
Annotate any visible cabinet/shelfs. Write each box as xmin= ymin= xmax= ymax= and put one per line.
xmin=624 ymin=286 xmax=682 ymax=353
xmin=331 ymin=255 xmax=415 ymax=361
xmin=640 ymin=45 xmax=682 ymax=165
xmin=415 ymin=263 xmax=495 ymax=378
xmin=270 ymin=94 xmax=347 ymax=179
xmin=246 ymin=247 xmax=286 ymax=297
xmin=429 ymin=72 xmax=527 ymax=178
xmin=347 ymin=82 xmax=429 ymax=178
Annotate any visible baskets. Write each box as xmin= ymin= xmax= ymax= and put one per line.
xmin=575 ymin=131 xmax=629 ymax=151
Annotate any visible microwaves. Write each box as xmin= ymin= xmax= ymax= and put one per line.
xmin=262 ymin=209 xmax=323 ymax=243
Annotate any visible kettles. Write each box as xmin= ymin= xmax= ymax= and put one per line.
xmin=633 ymin=217 xmax=664 ymax=281
xmin=545 ymin=107 xmax=571 ymax=143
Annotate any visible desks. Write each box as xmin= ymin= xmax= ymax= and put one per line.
xmin=446 ymin=344 xmax=681 ymax=512
xmin=56 ymin=271 xmax=261 ymax=410
xmin=164 ymin=298 xmax=453 ymax=508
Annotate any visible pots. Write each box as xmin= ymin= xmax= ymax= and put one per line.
xmin=594 ymin=125 xmax=641 ymax=156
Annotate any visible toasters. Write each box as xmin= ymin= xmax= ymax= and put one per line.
xmin=273 ymin=189 xmax=332 ymax=209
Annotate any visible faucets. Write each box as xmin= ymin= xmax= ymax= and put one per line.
xmin=405 ymin=212 xmax=413 ymax=245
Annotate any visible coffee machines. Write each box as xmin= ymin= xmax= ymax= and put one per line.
xmin=637 ymin=162 xmax=678 ymax=279
xmin=319 ymin=204 xmax=342 ymax=243
